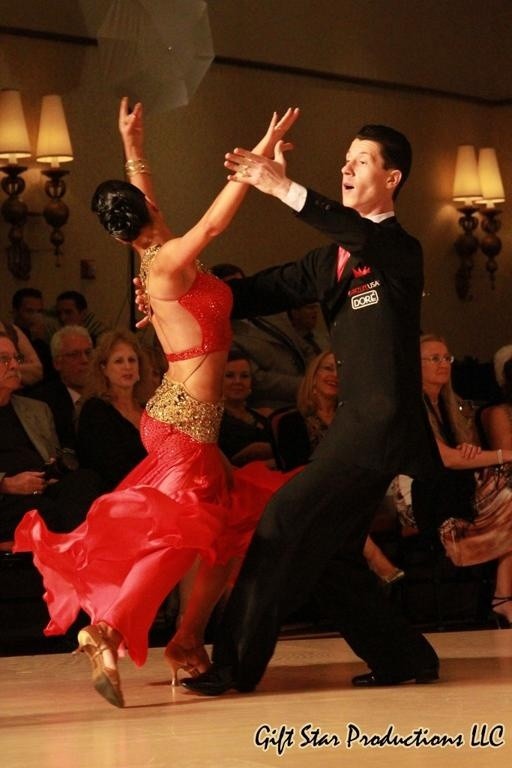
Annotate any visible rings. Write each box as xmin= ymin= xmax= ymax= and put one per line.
xmin=242 ymin=165 xmax=249 ymax=177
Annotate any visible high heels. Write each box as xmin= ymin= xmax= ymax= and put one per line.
xmin=367 ymin=557 xmax=406 ymax=588
xmin=488 ymin=595 xmax=512 ymax=628
xmin=163 ymin=636 xmax=211 ymax=688
xmin=71 ymin=621 xmax=127 ymax=710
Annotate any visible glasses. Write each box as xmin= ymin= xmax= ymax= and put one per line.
xmin=1 ymin=352 xmax=25 ymax=368
xmin=420 ymin=353 xmax=455 ymax=364
xmin=55 ymin=348 xmax=97 ymax=362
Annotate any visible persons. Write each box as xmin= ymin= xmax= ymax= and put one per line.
xmin=213 ymin=264 xmax=317 ymax=401
xmin=278 ymin=349 xmax=406 ymax=582
xmin=481 ymin=348 xmax=512 ymax=451
xmin=179 ymin=123 xmax=444 ymax=698
xmin=216 ymin=343 xmax=274 ymax=468
xmin=290 ymin=298 xmax=330 ymax=361
xmin=415 ymin=331 xmax=512 ymax=627
xmin=0 ymin=285 xmax=162 ymax=544
xmin=13 ymin=93 xmax=300 ymax=707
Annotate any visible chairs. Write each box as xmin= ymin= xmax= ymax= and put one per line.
xmin=474 ymin=402 xmax=494 ymax=450
xmin=389 ymin=530 xmax=475 ymax=618
xmin=270 ymin=409 xmax=312 ymax=468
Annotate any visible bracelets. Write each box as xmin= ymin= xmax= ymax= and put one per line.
xmin=497 ymin=449 xmax=503 ymax=464
xmin=123 ymin=158 xmax=151 ymax=179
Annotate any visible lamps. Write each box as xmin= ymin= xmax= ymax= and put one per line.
xmin=477 ymin=147 xmax=507 ymax=290
xmin=0 ymin=90 xmax=32 ymax=280
xmin=453 ymin=145 xmax=483 ymax=299
xmin=36 ymin=94 xmax=73 ymax=269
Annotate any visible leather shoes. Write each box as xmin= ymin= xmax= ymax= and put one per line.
xmin=349 ymin=655 xmax=442 ymax=686
xmin=178 ymin=661 xmax=260 ymax=695
